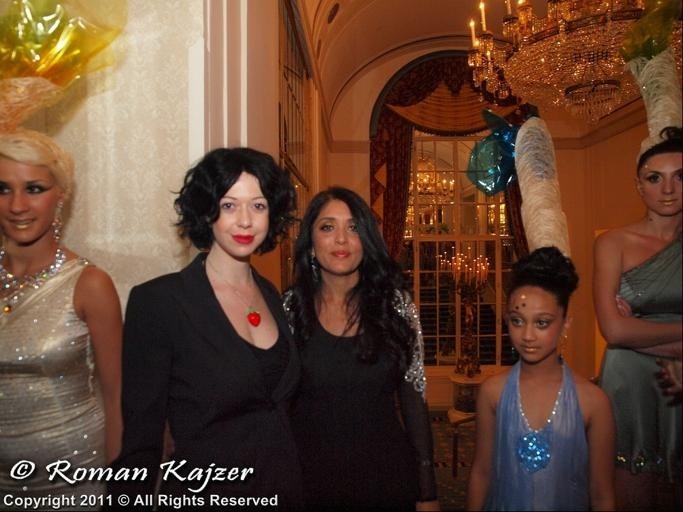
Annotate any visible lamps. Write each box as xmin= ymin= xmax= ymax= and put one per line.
xmin=439 ymin=247 xmax=488 ymax=377
xmin=435 ymin=252 xmax=450 ymax=278
xmin=404 ymin=130 xmax=455 ymax=225
xmin=465 ymin=0 xmax=683 ymax=127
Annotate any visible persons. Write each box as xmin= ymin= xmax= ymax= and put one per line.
xmin=97 ymin=144 xmax=318 ymax=510
xmin=655 ymin=356 xmax=682 ymax=395
xmin=462 ymin=244 xmax=621 ymax=511
xmin=263 ymin=180 xmax=446 ymax=511
xmin=589 ymin=120 xmax=683 ymax=512
xmin=0 ymin=124 xmax=127 ymax=509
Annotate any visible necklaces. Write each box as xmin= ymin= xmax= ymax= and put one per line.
xmin=0 ymin=241 xmax=64 ymax=317
xmin=205 ymin=258 xmax=262 ymax=327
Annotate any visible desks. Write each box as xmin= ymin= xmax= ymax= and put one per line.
xmin=435 ymin=348 xmax=463 ymax=366
xmin=447 ymin=409 xmax=477 ymax=478
